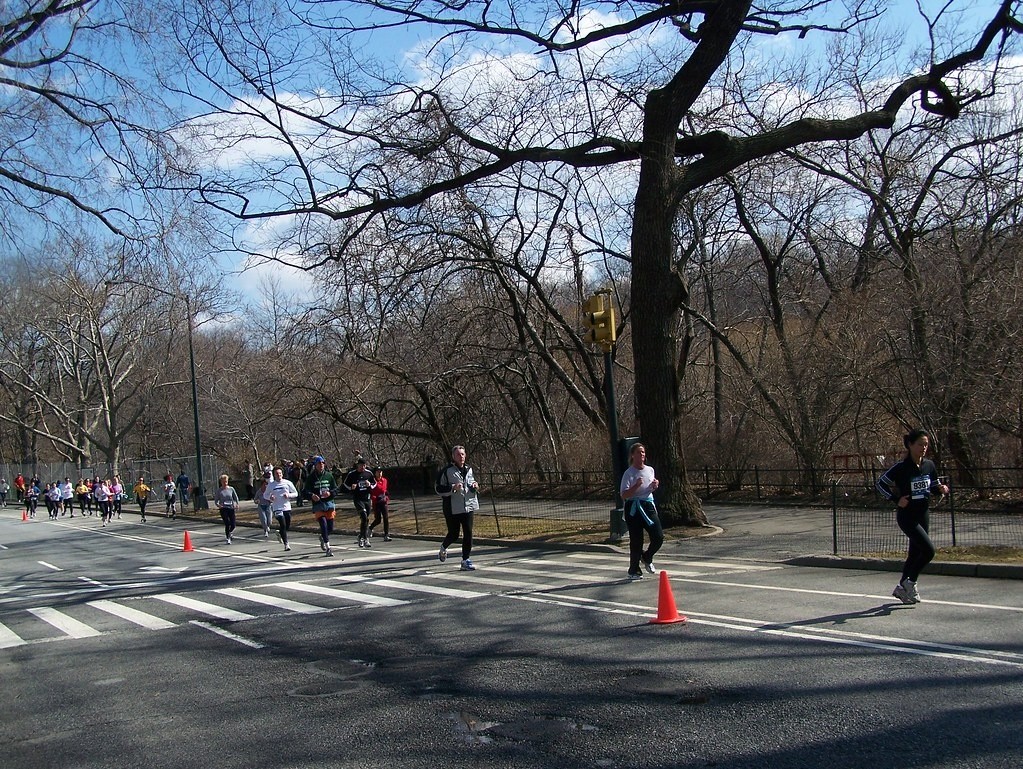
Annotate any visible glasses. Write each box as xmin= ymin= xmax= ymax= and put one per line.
xmin=318 ymin=462 xmax=325 ymax=464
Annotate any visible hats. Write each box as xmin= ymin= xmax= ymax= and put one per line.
xmin=374 ymin=466 xmax=383 ymax=472
xmin=313 ymin=457 xmax=325 ymax=465
xmin=357 ymin=459 xmax=366 ymax=464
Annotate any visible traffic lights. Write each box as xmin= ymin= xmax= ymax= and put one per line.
xmin=581 ymin=296 xmax=616 ymax=351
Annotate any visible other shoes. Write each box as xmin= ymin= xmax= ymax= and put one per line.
xmin=267 ymin=526 xmax=270 ymax=532
xmin=230 ymin=531 xmax=234 ymax=539
xmin=264 ymin=532 xmax=268 ymax=537
xmin=226 ymin=538 xmax=231 ymax=545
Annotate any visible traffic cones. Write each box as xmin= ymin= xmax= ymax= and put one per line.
xmin=182 ymin=530 xmax=194 ymax=552
xmin=649 ymin=570 xmax=688 ymax=624
xmin=22 ymin=510 xmax=27 ymax=521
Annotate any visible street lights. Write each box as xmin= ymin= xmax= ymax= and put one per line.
xmin=105 ymin=280 xmax=209 ymax=510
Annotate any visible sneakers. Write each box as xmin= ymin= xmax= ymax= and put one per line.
xmin=627 ymin=572 xmax=643 ymax=580
xmin=363 ymin=537 xmax=372 ymax=548
xmin=367 ymin=525 xmax=373 ymax=538
xmin=321 ymin=536 xmax=326 ymax=550
xmin=641 ymin=557 xmax=655 ymax=574
xmin=892 ymin=585 xmax=916 ymax=605
xmin=285 ymin=542 xmax=291 ymax=551
xmin=902 ymin=577 xmax=921 ymax=603
xmin=439 ymin=544 xmax=447 ymax=562
xmin=357 ymin=534 xmax=364 ymax=547
xmin=384 ymin=537 xmax=392 ymax=541
xmin=461 ymin=559 xmax=475 ymax=571
xmin=326 ymin=548 xmax=334 ymax=557
xmin=275 ymin=530 xmax=283 ymax=544
xmin=25 ymin=510 xmax=176 ymax=527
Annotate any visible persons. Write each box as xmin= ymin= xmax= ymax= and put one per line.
xmin=24 ymin=475 xmax=126 ymax=527
xmin=434 ymin=445 xmax=480 ymax=571
xmin=875 ymin=429 xmax=948 ymax=604
xmin=254 ymin=479 xmax=275 ymax=537
xmin=14 ymin=473 xmax=26 ymax=502
xmin=164 ymin=474 xmax=177 ymax=520
xmin=264 ymin=461 xmax=274 ymax=484
xmin=0 ymin=478 xmax=9 ymax=507
xmin=263 ymin=467 xmax=298 ymax=551
xmin=281 ymin=455 xmax=315 ymax=507
xmin=241 ymin=459 xmax=255 ymax=501
xmin=31 ymin=473 xmax=41 ymax=502
xmin=620 ymin=443 xmax=663 ymax=580
xmin=175 ymin=470 xmax=190 ymax=506
xmin=368 ymin=466 xmax=392 ymax=542
xmin=214 ymin=474 xmax=239 ymax=544
xmin=133 ymin=477 xmax=151 ymax=523
xmin=342 ymin=458 xmax=377 ymax=548
xmin=303 ymin=455 xmax=338 ymax=557
